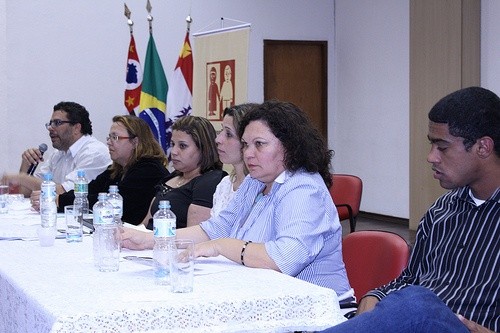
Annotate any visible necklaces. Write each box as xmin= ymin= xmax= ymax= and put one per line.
xmin=176 ymin=181 xmax=181 ymax=187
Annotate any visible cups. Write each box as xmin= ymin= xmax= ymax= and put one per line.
xmin=168 ymin=239 xmax=195 ymax=293
xmin=0 ymin=184 xmax=8 ymax=214
xmin=99 ymin=224 xmax=121 ymax=272
xmin=36 ymin=213 xmax=56 ymax=246
xmin=64 ymin=205 xmax=83 ymax=243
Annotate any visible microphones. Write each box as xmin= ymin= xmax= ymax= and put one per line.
xmin=26 ymin=144 xmax=48 ymax=176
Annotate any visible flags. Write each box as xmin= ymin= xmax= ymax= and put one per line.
xmin=139 ymin=34 xmax=169 ymax=163
xmin=124 ymin=36 xmax=144 ymax=117
xmin=165 ymin=32 xmax=194 ymax=165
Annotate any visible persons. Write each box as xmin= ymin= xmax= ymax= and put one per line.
xmin=318 ymin=86 xmax=500 ymax=333
xmin=135 ymin=116 xmax=229 ymax=231
xmin=30 ymin=115 xmax=171 ymax=226
xmin=0 ymin=102 xmax=112 ymax=197
xmin=210 ymin=103 xmax=261 ymax=220
xmin=112 ymin=98 xmax=356 ymax=308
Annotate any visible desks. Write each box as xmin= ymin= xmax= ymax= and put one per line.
xmin=0 ymin=193 xmax=339 ymax=333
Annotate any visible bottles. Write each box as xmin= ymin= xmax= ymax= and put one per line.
xmin=106 ymin=185 xmax=123 ymax=261
xmin=93 ymin=193 xmax=115 ymax=264
xmin=152 ymin=201 xmax=176 ymax=279
xmin=39 ymin=173 xmax=57 ymax=228
xmin=73 ymin=171 xmax=90 ymax=217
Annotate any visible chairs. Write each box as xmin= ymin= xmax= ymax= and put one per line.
xmin=342 ymin=229 xmax=410 ymax=304
xmin=331 ymin=173 xmax=362 ymax=232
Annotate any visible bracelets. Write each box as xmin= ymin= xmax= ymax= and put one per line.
xmin=241 ymin=241 xmax=253 ymax=266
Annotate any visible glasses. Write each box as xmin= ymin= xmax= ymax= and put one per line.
xmin=45 ymin=118 xmax=74 ymax=129
xmin=105 ymin=133 xmax=137 ymax=142
